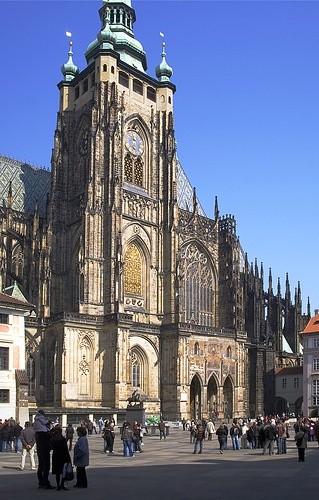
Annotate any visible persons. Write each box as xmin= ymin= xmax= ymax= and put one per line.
xmin=0 ymin=410 xmax=148 ymax=491
xmin=182 ymin=412 xmax=319 ymax=463
xmin=158 ymin=419 xmax=167 ymax=440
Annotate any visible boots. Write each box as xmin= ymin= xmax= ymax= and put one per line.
xmin=60 ymin=478 xmax=69 ymax=490
xmin=56 ymin=478 xmax=60 ymax=490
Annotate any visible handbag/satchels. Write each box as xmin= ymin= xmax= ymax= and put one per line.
xmin=295 ymin=438 xmax=303 ymax=445
xmin=64 ymin=463 xmax=75 ymax=481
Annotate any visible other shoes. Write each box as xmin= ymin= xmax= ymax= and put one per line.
xmin=39 ymin=484 xmax=44 ymax=488
xmin=31 ymin=468 xmax=37 ymax=471
xmin=73 ymin=484 xmax=78 ymax=487
xmin=18 ymin=467 xmax=24 ymax=470
xmin=102 ymin=447 xmax=143 ymax=457
xmin=160 ymin=438 xmax=167 ymax=440
xmin=77 ymin=485 xmax=87 ymax=488
xmin=45 ymin=484 xmax=57 ymax=489
